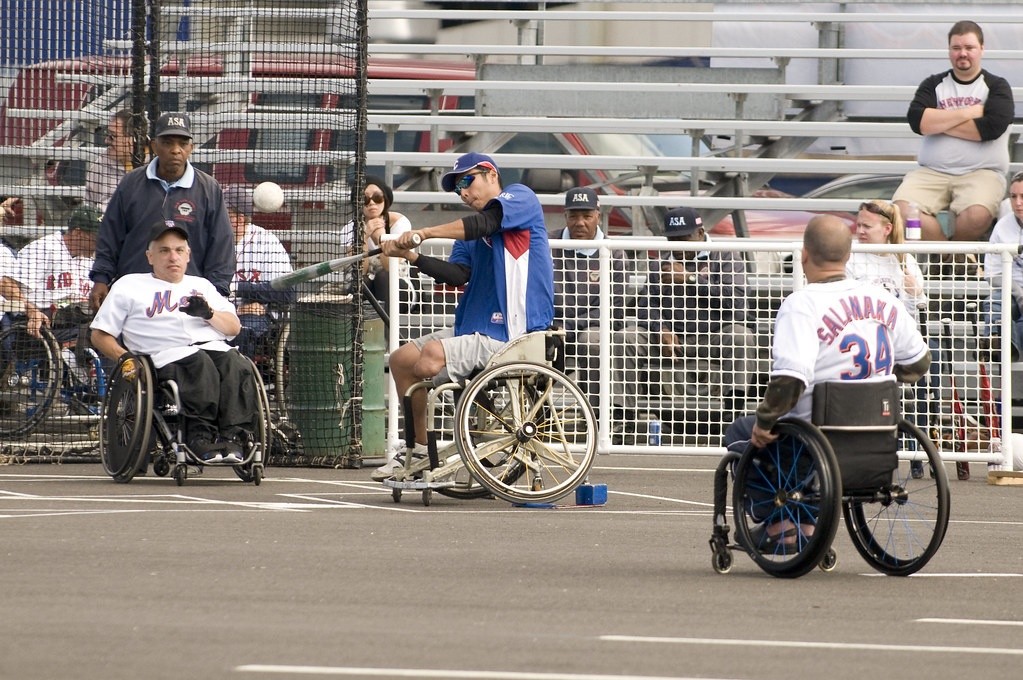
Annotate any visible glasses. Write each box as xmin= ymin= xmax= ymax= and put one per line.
xmin=454 ymin=170 xmax=488 ymax=196
xmin=361 ymin=193 xmax=383 ymax=206
xmin=104 ymin=129 xmax=133 ymax=142
xmin=858 ymin=202 xmax=891 ymax=219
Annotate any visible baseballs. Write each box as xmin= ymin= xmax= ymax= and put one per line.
xmin=252 ymin=182 xmax=286 ymax=213
xmin=903 ymin=302 xmax=1000 ymax=481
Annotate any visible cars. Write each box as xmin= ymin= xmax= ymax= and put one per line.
xmin=1 ymin=60 xmax=1023 ymax=434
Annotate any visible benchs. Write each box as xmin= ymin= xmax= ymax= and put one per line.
xmin=0 ymin=7 xmax=1023 ymax=422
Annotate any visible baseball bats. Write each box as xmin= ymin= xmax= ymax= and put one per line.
xmin=269 ymin=232 xmax=422 ymax=290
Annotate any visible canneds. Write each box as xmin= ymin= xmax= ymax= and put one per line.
xmin=648 ymin=421 xmax=662 ymax=445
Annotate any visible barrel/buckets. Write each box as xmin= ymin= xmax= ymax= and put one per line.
xmin=290 ymin=300 xmax=387 ymax=458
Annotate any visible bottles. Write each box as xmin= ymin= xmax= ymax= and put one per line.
xmin=906 ymin=202 xmax=922 ymax=241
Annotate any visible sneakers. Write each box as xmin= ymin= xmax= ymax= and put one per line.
xmin=220 ymin=436 xmax=243 ymax=463
xmin=190 ymin=439 xmax=223 ymax=464
xmin=371 ymin=443 xmax=429 ymax=482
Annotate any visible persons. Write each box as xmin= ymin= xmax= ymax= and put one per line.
xmin=0 ymin=196 xmax=103 ymax=362
xmin=85 ymin=109 xmax=156 ymax=213
xmin=221 ymin=186 xmax=293 ymax=363
xmin=341 ymin=175 xmax=426 ymax=313
xmin=891 ymin=21 xmax=1015 ymax=272
xmin=724 ymin=216 xmax=931 ymax=553
xmin=89 ymin=111 xmax=235 ymax=317
xmin=89 ymin=220 xmax=257 ymax=463
xmin=547 ymin=186 xmax=630 ymax=421
xmin=847 ymin=201 xmax=941 ymax=436
xmin=983 ymin=171 xmax=1023 ymax=363
xmin=615 ymin=207 xmax=757 ymax=430
xmin=372 ymin=152 xmax=552 ymax=480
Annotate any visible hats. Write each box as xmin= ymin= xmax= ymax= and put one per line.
xmin=155 ymin=113 xmax=194 ymax=141
xmin=662 ymin=206 xmax=703 ymax=237
xmin=350 ymin=177 xmax=393 ymax=210
xmin=441 ymin=151 xmax=501 ymax=193
xmin=68 ymin=206 xmax=105 ymax=232
xmin=222 ymin=187 xmax=255 ymax=216
xmin=564 ymin=186 xmax=599 ymax=211
xmin=146 ymin=222 xmax=189 ymax=249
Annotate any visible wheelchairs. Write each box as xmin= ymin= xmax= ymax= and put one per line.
xmin=384 ymin=326 xmax=598 ymax=507
xmin=99 ymin=350 xmax=273 ymax=487
xmin=709 ymin=375 xmax=952 ymax=578
xmin=0 ymin=321 xmax=105 ymax=441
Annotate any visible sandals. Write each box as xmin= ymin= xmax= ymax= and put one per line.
xmin=733 ymin=523 xmax=798 ymax=556
xmin=800 ymin=536 xmax=812 ymax=548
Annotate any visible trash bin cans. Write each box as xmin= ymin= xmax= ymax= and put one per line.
xmin=288 ymin=299 xmax=387 ymax=467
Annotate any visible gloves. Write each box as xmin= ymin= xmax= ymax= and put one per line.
xmin=179 ymin=296 xmax=214 ymax=320
